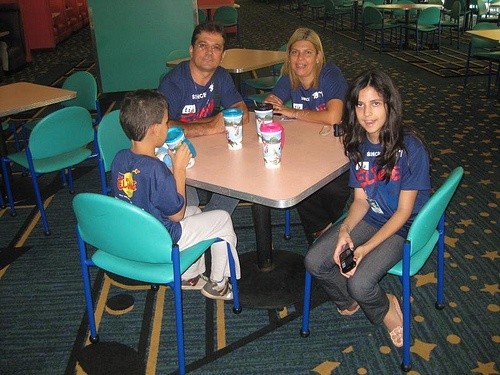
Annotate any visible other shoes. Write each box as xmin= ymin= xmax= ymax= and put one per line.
xmin=384 ymin=294 xmax=405 ymax=348
xmin=337 ymin=302 xmax=361 ymax=316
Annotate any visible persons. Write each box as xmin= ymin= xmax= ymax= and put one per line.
xmin=158 ymin=22 xmax=250 ymax=139
xmin=304 ymin=67 xmax=432 ymax=348
xmin=261 ymin=27 xmax=350 ymax=238
xmin=109 ymin=88 xmax=241 ymax=300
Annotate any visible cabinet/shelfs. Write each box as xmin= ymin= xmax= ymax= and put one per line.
xmin=1 ymin=0 xmax=89 ymax=49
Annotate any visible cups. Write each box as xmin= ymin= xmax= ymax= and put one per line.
xmin=259 ymin=122 xmax=284 ymax=170
xmin=254 ymin=102 xmax=273 ymax=144
xmin=164 ymin=126 xmax=196 ymax=170
xmin=222 ymin=107 xmax=244 ymax=150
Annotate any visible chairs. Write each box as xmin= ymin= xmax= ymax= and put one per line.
xmin=0 ymin=0 xmax=500 ymax=375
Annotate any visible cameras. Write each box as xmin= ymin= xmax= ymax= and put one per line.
xmin=333 ymin=123 xmax=344 ymax=137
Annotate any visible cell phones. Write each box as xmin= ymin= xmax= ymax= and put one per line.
xmin=339 ymin=248 xmax=356 ymax=274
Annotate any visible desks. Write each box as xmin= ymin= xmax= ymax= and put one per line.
xmin=166 ymin=48 xmax=288 ymax=94
xmin=372 ymin=4 xmax=442 ymax=50
xmin=199 ymin=5 xmax=218 ymax=24
xmin=463 ymin=29 xmax=500 ymax=97
xmin=0 ymin=82 xmax=78 ymax=193
xmin=170 ymin=112 xmax=354 ymax=309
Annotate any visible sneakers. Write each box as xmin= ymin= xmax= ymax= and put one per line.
xmin=201 ymin=276 xmax=235 ymax=301
xmin=181 ymin=273 xmax=209 ymax=290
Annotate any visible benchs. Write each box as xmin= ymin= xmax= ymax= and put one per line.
xmin=0 ymin=3 xmax=27 ymax=75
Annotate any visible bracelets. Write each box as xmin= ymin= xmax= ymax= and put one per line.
xmin=295 ymin=109 xmax=310 ymax=120
xmin=338 ymin=224 xmax=352 ymax=233
